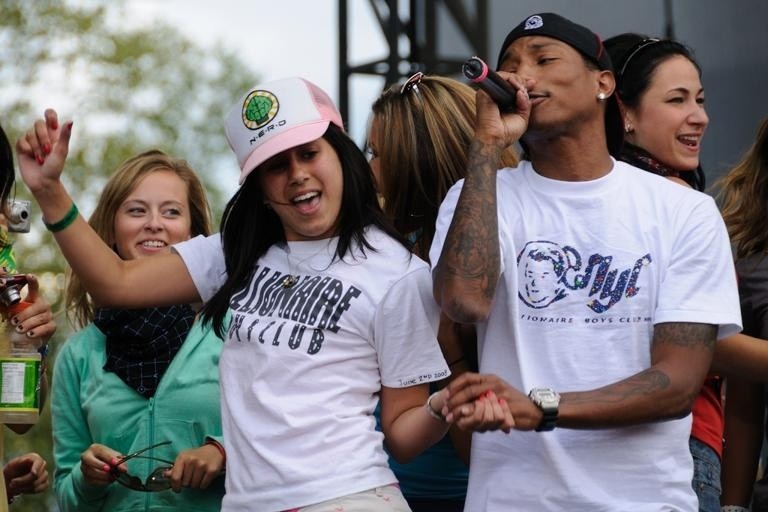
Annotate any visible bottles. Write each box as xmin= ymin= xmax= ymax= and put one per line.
xmin=0 ymin=301 xmax=43 ymax=425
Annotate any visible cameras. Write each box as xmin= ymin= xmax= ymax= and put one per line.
xmin=0 ymin=273 xmax=29 ymax=307
xmin=6 ymin=198 xmax=32 ymax=233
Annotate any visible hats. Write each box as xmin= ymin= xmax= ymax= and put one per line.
xmin=223 ymin=77 xmax=344 ymax=185
xmin=496 ymin=13 xmax=616 ymax=77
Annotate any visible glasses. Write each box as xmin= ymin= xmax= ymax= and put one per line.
xmin=110 ymin=441 xmax=177 ymax=492
xmin=401 ymin=71 xmax=428 ymax=115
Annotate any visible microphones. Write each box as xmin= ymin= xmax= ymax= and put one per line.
xmin=461 ymin=56 xmax=518 ymax=112
